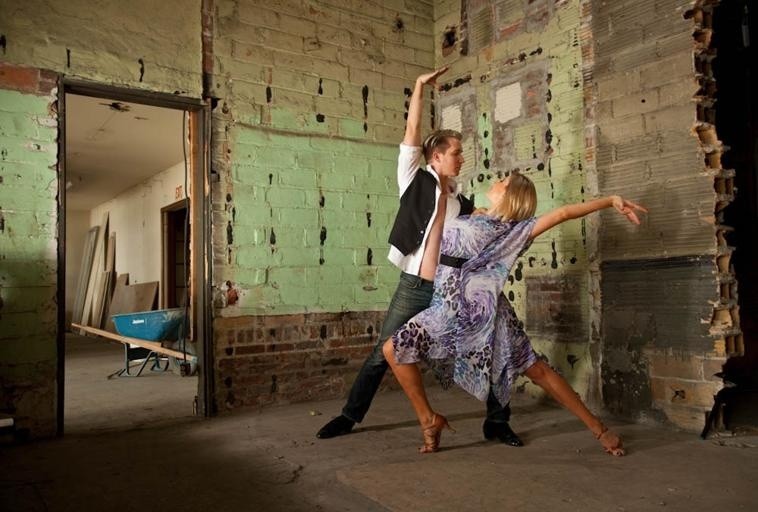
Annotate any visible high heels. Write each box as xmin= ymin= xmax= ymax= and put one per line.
xmin=419 ymin=412 xmax=457 ymax=452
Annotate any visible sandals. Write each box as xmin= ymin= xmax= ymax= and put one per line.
xmin=595 ymin=425 xmax=627 ymax=458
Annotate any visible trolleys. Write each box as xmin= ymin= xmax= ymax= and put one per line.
xmin=71 ymin=308 xmax=197 ymax=377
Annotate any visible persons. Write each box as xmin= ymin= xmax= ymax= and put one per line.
xmin=381 ymin=172 xmax=648 ymax=452
xmin=316 ymin=67 xmax=524 ymax=447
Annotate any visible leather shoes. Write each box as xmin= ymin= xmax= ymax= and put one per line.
xmin=482 ymin=420 xmax=524 ymax=448
xmin=316 ymin=414 xmax=356 ymax=439
